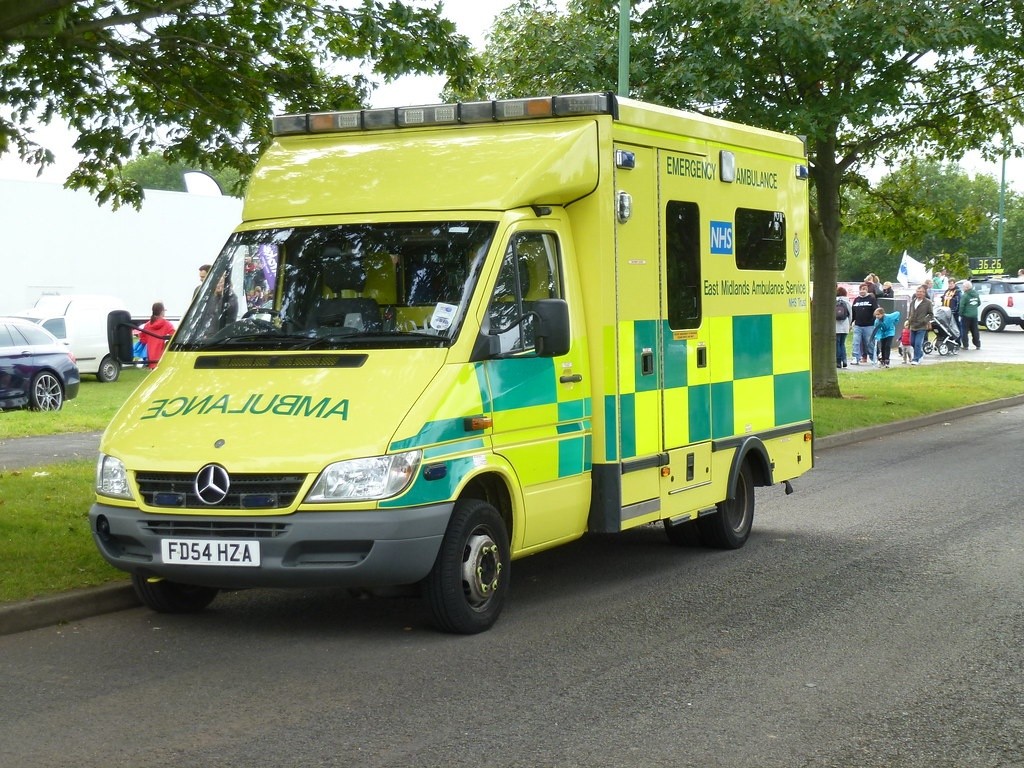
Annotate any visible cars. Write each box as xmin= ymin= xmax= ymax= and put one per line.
xmin=0 ymin=316 xmax=81 ymax=414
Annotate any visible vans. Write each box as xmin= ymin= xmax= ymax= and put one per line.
xmin=1 ymin=282 xmax=131 ymax=383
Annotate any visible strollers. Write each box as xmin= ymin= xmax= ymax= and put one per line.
xmin=922 ymin=306 xmax=963 ymax=354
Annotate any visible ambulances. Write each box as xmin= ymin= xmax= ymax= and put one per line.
xmin=89 ymin=90 xmax=812 ymax=638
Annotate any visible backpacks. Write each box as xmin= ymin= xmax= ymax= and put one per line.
xmin=836 ymin=299 xmax=850 ymax=320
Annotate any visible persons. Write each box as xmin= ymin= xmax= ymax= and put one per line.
xmin=835 ymin=269 xmax=981 ymax=368
xmin=1016 ymin=269 xmax=1024 ymax=278
xmin=244 ymin=257 xmax=275 ymax=308
xmin=192 ymin=265 xmax=238 ymax=332
xmin=138 ymin=303 xmax=175 ymax=369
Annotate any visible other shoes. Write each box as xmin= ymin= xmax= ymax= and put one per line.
xmin=860 ymin=357 xmax=867 ymax=362
xmin=837 ymin=363 xmax=842 ymax=368
xmin=851 ymin=358 xmax=857 ymax=365
xmin=898 ymin=346 xmax=902 ymax=355
xmin=910 ymin=359 xmax=920 ymax=365
xmin=843 ymin=362 xmax=847 ymax=367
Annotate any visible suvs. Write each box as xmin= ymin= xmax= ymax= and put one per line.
xmin=837 ymin=275 xmax=1024 ymax=333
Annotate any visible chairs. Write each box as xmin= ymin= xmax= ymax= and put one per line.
xmin=452 ymin=254 xmax=531 ymax=331
xmin=305 ymin=254 xmax=384 ymax=334
